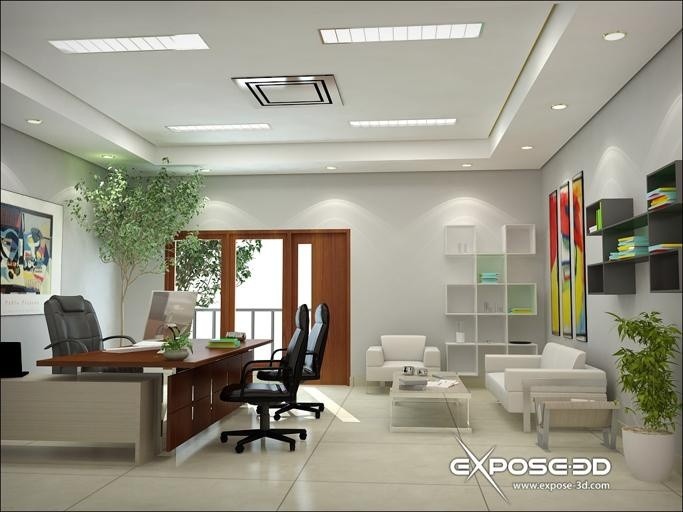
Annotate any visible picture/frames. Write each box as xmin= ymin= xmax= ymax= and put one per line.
xmin=559 ymin=181 xmax=573 ymax=339
xmin=549 ymin=190 xmax=560 ymax=336
xmin=572 ymin=171 xmax=588 ymax=343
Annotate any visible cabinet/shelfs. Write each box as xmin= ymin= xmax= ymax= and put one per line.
xmin=443 ymin=222 xmax=539 ymax=377
xmin=586 ymin=160 xmax=683 ymax=295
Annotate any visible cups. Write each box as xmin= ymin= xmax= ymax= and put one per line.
xmin=483 ymin=302 xmax=488 ymax=313
xmin=417 ymin=368 xmax=428 ymax=376
xmin=403 ymin=366 xmax=414 ymax=375
xmin=495 ymin=303 xmax=503 ymax=312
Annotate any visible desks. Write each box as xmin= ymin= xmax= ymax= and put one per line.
xmin=0 ymin=373 xmax=163 ymax=465
xmin=36 ymin=338 xmax=272 ymax=450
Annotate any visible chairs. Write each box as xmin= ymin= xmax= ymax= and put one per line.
xmin=220 ymin=303 xmax=310 ymax=454
xmin=44 ymin=295 xmax=136 ymax=374
xmin=365 ymin=335 xmax=442 ymax=389
xmin=257 ymin=303 xmax=331 ymax=420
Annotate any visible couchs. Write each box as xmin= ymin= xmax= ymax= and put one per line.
xmin=485 ymin=342 xmax=607 ymax=434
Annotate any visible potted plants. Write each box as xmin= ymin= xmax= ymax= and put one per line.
xmin=606 ymin=310 xmax=683 ymax=484
xmin=158 ymin=320 xmax=193 ymax=360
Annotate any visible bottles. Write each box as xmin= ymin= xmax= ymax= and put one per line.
xmin=455 ymin=318 xmax=465 ymax=342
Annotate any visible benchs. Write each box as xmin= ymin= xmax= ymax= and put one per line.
xmin=389 ymin=372 xmax=472 ymax=434
xmin=528 ymin=390 xmax=620 ymax=451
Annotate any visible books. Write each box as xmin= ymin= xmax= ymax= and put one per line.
xmin=645 ymin=188 xmax=676 ymax=210
xmin=206 ymin=337 xmax=240 ymax=348
xmin=510 ymin=307 xmax=533 ymax=314
xmin=427 ymin=379 xmax=460 ymax=388
xmin=648 ymin=244 xmax=682 ymax=252
xmin=609 ymin=236 xmax=649 ymax=259
xmin=596 ymin=209 xmax=602 ymax=230
